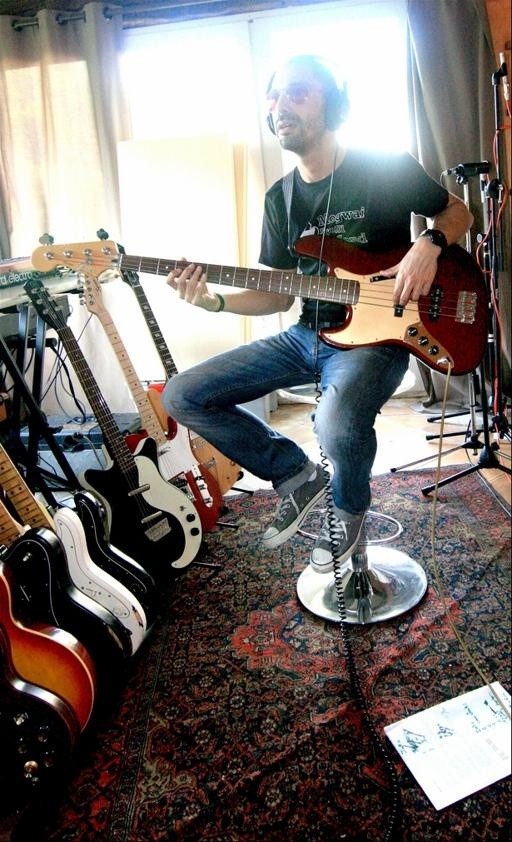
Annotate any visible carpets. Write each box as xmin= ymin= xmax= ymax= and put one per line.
xmin=0 ymin=461 xmax=512 ymax=841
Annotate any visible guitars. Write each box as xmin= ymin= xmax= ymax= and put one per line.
xmin=31 ymin=231 xmax=492 ymax=375
xmin=0 ymin=230 xmax=242 ymax=791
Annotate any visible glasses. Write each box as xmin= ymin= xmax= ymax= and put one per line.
xmin=260 ymin=80 xmax=333 ymax=113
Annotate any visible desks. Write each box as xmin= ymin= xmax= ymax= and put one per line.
xmin=1 ymin=270 xmax=117 ymax=498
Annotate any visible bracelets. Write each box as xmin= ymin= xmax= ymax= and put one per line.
xmin=212 ymin=292 xmax=226 ymax=313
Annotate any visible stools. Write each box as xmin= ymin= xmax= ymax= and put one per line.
xmin=275 ymin=369 xmax=428 ymax=627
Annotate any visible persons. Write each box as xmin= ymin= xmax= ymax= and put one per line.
xmin=159 ymin=52 xmax=476 ymax=574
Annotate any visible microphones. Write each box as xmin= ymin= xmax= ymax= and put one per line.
xmin=443 ymin=160 xmax=492 ymax=178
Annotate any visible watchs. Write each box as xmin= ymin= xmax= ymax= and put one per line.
xmin=418 ymin=227 xmax=450 ymax=256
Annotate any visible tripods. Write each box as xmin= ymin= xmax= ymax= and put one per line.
xmin=391 ymin=181 xmax=511 ymax=496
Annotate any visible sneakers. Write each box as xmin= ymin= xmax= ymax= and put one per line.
xmin=260 ymin=462 xmax=334 ymax=548
xmin=310 ymin=505 xmax=375 ymax=573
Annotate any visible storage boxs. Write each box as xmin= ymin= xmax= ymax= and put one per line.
xmin=19 ymin=414 xmax=141 ymax=482
